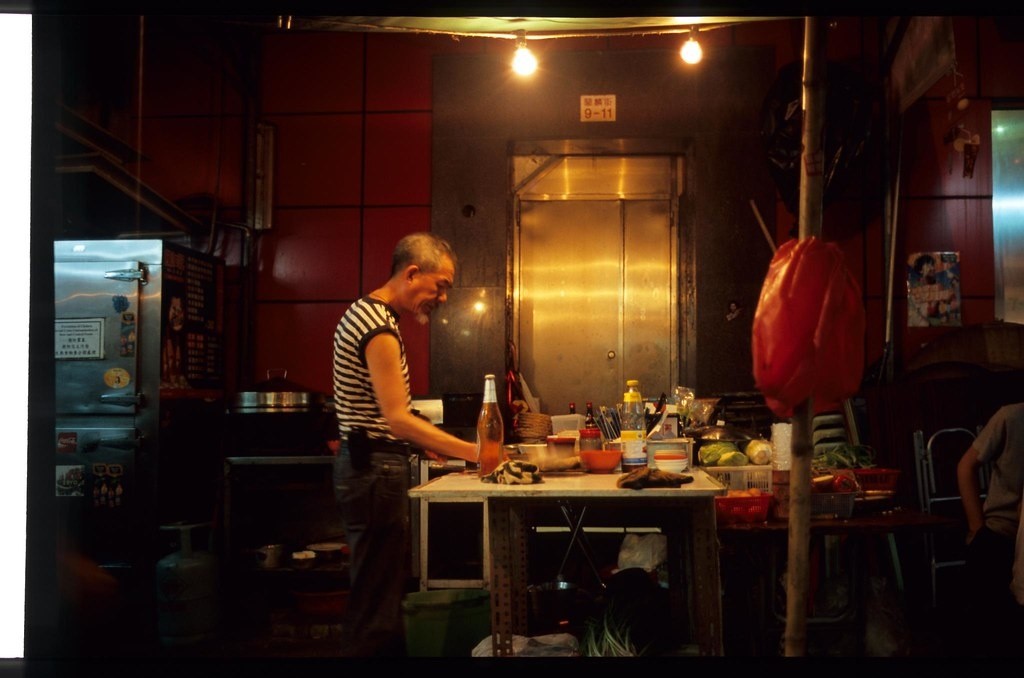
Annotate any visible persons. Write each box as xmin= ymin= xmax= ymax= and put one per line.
xmin=909 ymin=256 xmax=960 ymax=326
xmin=333 ymin=233 xmax=513 ymax=658
xmin=955 ymin=402 xmax=1024 ymax=657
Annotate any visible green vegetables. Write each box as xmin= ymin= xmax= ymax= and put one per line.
xmin=814 ymin=443 xmax=878 ymax=469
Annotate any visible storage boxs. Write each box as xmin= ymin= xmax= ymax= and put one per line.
xmin=703 ymin=463 xmax=900 ymax=519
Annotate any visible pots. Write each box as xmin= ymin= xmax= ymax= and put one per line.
xmin=224 ymin=368 xmax=335 ymax=456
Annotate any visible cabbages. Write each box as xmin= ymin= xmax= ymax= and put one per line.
xmin=697 ymin=436 xmax=773 ymax=467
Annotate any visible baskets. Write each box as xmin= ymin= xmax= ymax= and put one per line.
xmin=715 ymin=490 xmax=773 ymax=524
xmin=773 ymin=492 xmax=856 ymax=523
xmin=516 ymin=412 xmax=552 ymax=444
xmin=837 ymin=469 xmax=901 ymax=490
xmin=699 ymin=463 xmax=772 ymax=493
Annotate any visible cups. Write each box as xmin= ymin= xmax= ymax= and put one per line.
xmin=579 ymin=429 xmax=601 ymax=450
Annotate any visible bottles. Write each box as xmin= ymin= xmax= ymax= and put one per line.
xmin=620 ymin=380 xmax=647 ymax=471
xmin=585 ymin=401 xmax=597 ymax=428
xmin=478 ymin=375 xmax=504 ymax=479
xmin=569 ymin=402 xmax=576 ymax=414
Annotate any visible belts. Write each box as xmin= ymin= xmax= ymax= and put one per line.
xmin=340 ymin=440 xmax=408 ymax=455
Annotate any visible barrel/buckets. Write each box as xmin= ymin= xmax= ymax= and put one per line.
xmin=400 ymin=589 xmax=490 ymax=657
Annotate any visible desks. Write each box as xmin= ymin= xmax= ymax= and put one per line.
xmin=717 ymin=506 xmax=958 ymax=654
xmin=407 ymin=469 xmax=727 ymax=656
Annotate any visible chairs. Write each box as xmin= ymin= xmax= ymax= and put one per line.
xmin=914 ymin=422 xmax=990 ymax=607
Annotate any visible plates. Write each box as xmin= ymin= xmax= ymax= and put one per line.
xmin=306 ymin=542 xmax=346 ymax=552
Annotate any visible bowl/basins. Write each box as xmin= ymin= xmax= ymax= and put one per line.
xmin=655 ymin=449 xmax=690 ymax=473
xmin=295 ymin=590 xmax=351 ymax=616
xmin=581 ymin=450 xmax=621 ymax=474
xmin=518 ymin=444 xmax=547 ymax=455
